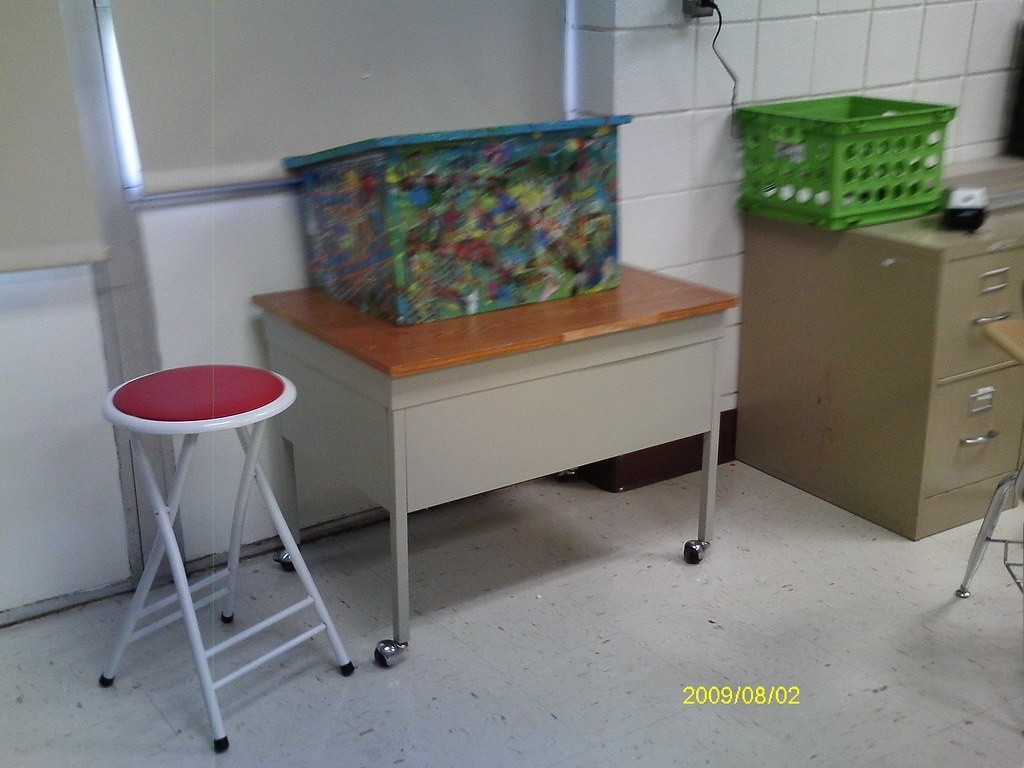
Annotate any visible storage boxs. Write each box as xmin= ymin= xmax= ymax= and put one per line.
xmin=282 ymin=115 xmax=632 ymax=326
xmin=735 ymin=96 xmax=959 ymax=231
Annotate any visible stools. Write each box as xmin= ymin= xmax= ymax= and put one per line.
xmin=99 ymin=364 xmax=355 ymax=754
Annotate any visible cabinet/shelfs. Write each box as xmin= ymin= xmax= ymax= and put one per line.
xmin=735 ymin=165 xmax=1024 ymax=542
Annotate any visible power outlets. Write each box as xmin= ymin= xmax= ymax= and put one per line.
xmin=683 ymin=0 xmax=714 ymax=18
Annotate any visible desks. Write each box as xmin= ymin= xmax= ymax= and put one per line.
xmin=251 ymin=264 xmax=740 ymax=668
